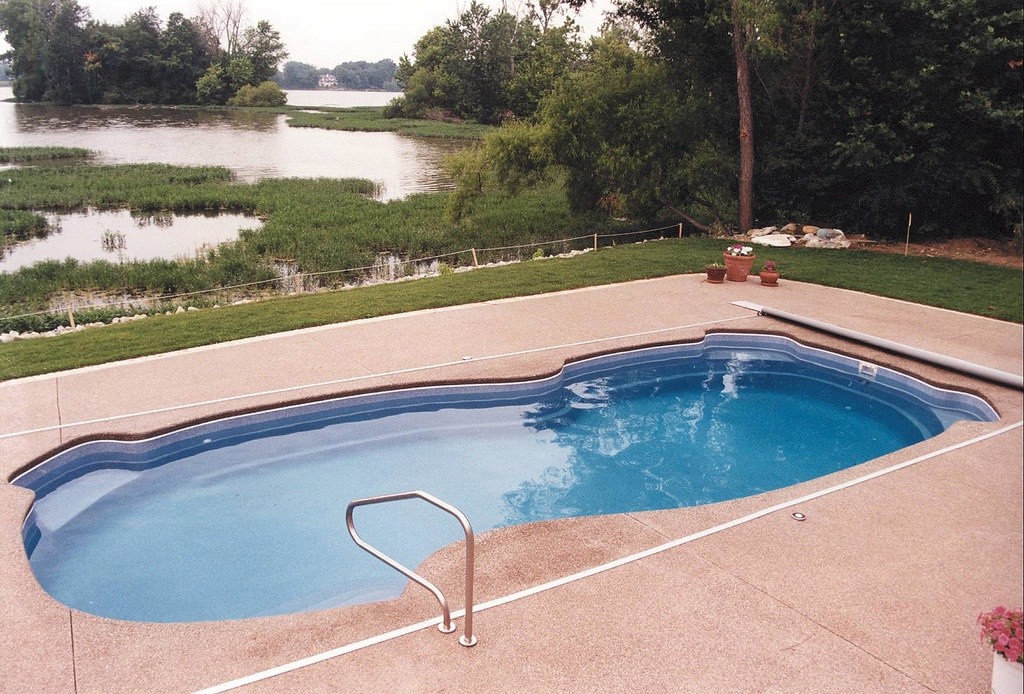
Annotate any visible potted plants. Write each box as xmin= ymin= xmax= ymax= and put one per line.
xmin=705 ymin=263 xmax=728 ymax=285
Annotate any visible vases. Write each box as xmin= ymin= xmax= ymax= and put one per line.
xmin=723 ymin=251 xmax=755 ymax=282
xmin=991 ymin=648 xmax=1024 ymax=694
xmin=760 ymin=270 xmax=779 ymax=287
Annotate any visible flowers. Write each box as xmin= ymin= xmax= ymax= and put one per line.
xmin=759 ymin=261 xmax=780 ymax=271
xmin=724 ymin=244 xmax=756 ymax=256
xmin=976 ymin=605 xmax=1024 ymax=661
